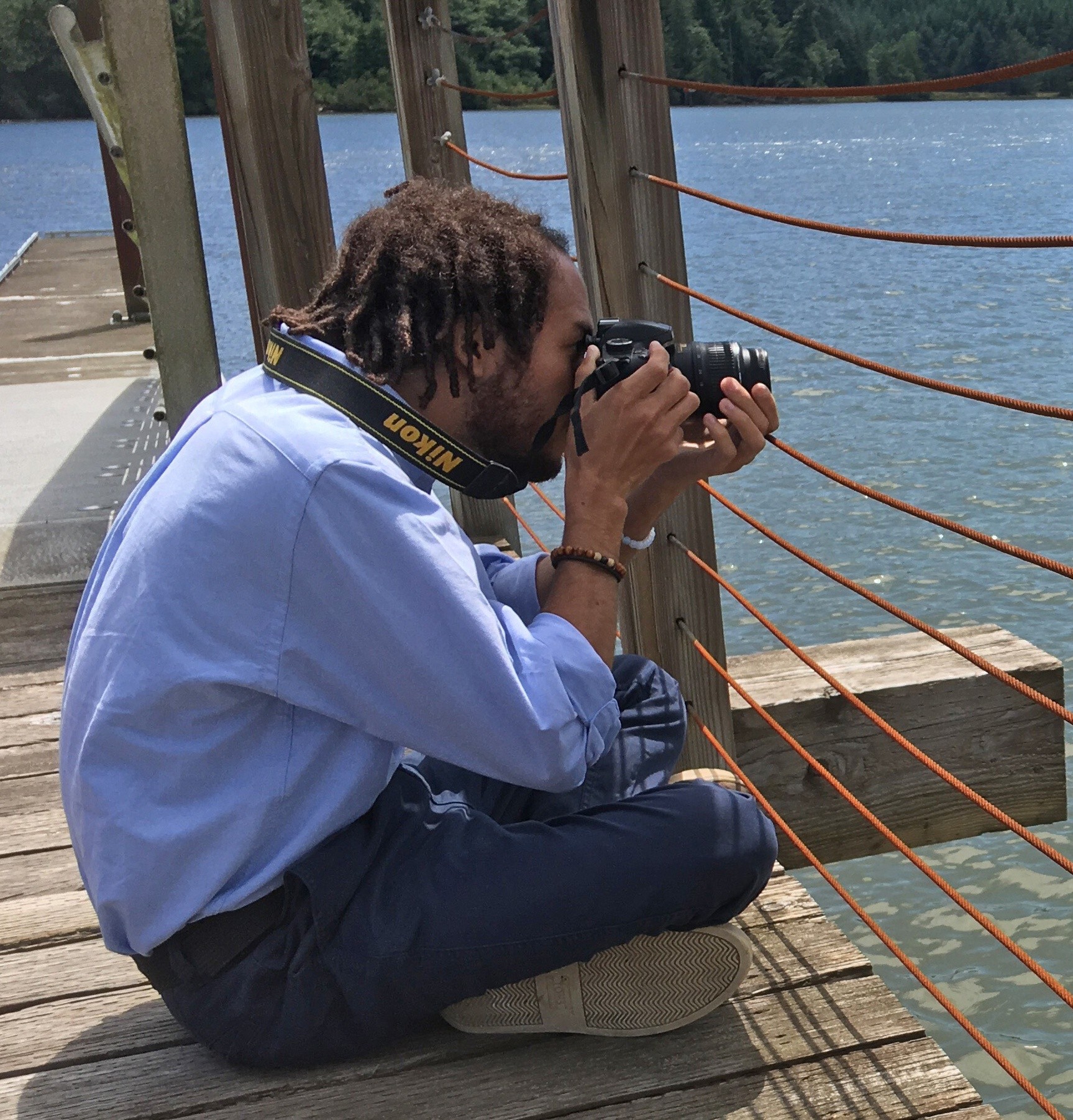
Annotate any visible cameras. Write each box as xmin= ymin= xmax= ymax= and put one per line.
xmin=589 ymin=317 xmax=773 ymax=419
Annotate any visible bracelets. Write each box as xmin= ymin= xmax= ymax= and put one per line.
xmin=621 ymin=527 xmax=656 ymax=550
xmin=550 ymin=546 xmax=627 ymax=583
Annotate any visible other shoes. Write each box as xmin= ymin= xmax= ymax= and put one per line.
xmin=668 ymin=768 xmax=750 ymax=793
xmin=440 ymin=922 xmax=754 ymax=1037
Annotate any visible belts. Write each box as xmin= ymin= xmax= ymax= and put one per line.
xmin=144 ymin=879 xmax=300 ymax=988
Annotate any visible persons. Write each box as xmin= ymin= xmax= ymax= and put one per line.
xmin=57 ymin=173 xmax=782 ymax=1066
